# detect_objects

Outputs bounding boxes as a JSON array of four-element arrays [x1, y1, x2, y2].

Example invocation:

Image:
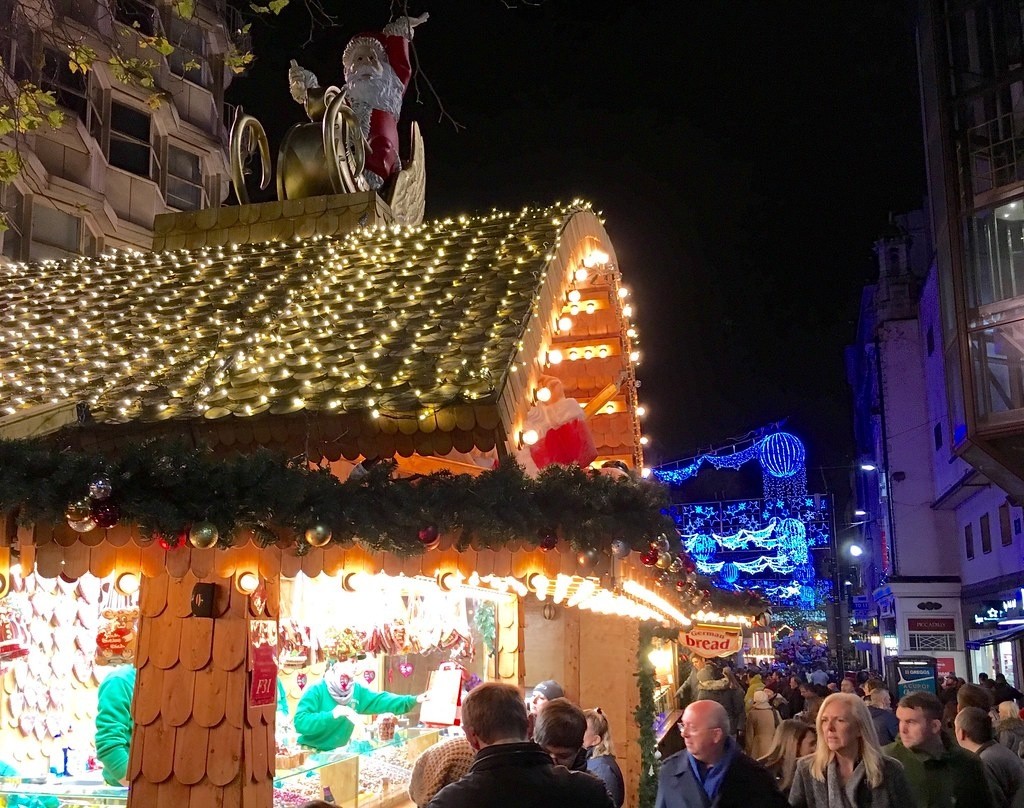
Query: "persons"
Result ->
[[405, 677, 625, 808], [651, 700, 794, 808], [292, 662, 432, 753], [672, 630, 1024, 755], [754, 718, 818, 798], [786, 693, 915, 808], [275, 674, 290, 754], [880, 690, 993, 808], [94, 666, 135, 788], [950, 707, 1024, 808]]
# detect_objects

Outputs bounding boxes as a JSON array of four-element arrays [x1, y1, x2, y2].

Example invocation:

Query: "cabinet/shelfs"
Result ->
[[0, 783, 129, 808], [273, 725, 467, 808], [652, 685, 676, 731]]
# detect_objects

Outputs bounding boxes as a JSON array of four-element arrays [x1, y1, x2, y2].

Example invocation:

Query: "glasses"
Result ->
[[677, 722, 723, 736]]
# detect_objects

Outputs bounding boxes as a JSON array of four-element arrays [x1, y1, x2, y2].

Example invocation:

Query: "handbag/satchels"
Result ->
[[419, 662, 464, 727]]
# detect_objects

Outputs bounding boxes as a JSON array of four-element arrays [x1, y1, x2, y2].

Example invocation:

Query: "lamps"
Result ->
[[529, 573, 549, 592], [442, 572, 460, 591], [345, 573, 363, 592], [517, 250, 653, 479], [119, 573, 138, 594], [240, 572, 259, 592]]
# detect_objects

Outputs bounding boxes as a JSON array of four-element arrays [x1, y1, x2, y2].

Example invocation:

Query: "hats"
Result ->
[[696, 665, 714, 683], [764, 681, 777, 695], [409, 737, 477, 808], [533, 680, 564, 702], [946, 675, 959, 682]]
